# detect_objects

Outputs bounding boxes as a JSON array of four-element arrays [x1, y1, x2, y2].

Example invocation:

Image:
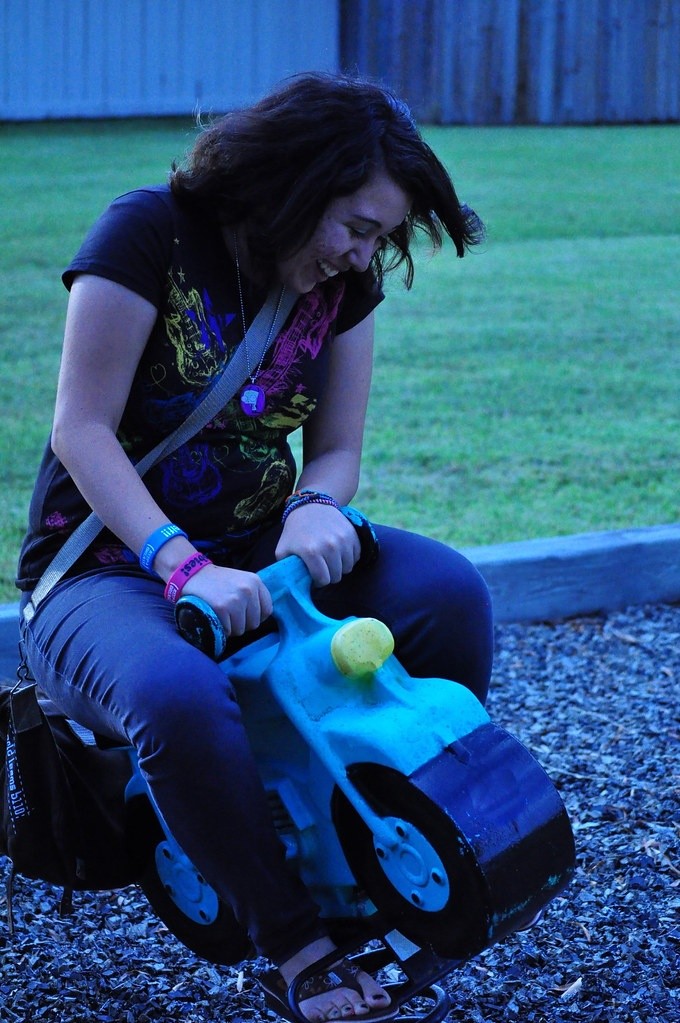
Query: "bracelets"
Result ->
[[281, 489, 339, 525], [139, 522, 188, 579], [164, 553, 213, 605]]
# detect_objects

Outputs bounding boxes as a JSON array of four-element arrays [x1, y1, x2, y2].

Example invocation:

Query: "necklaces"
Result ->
[[234, 235, 288, 418]]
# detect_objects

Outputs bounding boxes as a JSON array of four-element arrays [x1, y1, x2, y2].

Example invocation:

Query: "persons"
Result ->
[[16, 72, 497, 1023]]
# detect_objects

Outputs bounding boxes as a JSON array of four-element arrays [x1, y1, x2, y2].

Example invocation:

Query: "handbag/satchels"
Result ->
[[0, 670, 140, 890]]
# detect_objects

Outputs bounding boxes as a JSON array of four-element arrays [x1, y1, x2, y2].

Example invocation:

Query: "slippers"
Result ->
[[256, 956, 399, 1023]]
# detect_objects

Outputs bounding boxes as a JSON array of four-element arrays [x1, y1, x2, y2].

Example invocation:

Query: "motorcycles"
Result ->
[[0, 505, 577, 1018]]
